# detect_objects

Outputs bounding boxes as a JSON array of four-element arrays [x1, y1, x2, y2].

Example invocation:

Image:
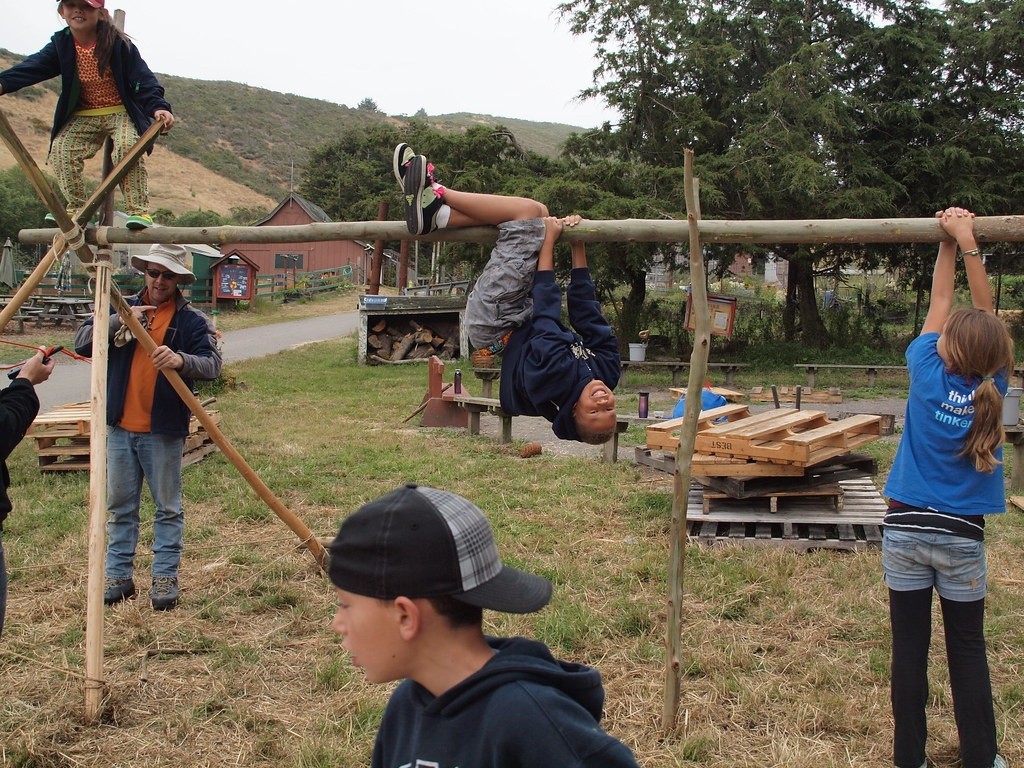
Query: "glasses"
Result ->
[[145, 268, 176, 280]]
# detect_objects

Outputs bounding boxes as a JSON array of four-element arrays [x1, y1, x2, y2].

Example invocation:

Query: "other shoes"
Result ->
[[895, 757, 927, 767], [961, 754, 1006, 768]]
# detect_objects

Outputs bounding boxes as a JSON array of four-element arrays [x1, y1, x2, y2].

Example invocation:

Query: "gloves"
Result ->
[[114, 321, 148, 346]]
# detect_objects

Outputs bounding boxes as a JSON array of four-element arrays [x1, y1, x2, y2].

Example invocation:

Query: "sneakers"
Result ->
[[152, 576, 178, 610], [392, 143, 415, 193], [45, 211, 76, 227], [405, 155, 446, 236], [104, 577, 135, 604], [126, 214, 154, 229]]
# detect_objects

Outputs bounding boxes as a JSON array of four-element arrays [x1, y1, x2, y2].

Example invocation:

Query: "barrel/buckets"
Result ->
[[1001, 388, 1022, 425], [629, 343, 646, 362]]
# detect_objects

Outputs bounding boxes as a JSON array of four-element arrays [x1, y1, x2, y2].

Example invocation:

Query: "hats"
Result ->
[[131, 244, 195, 285], [329, 483, 552, 614], [56, 0, 105, 8]]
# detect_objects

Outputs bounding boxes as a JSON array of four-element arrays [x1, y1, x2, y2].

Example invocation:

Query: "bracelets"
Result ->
[[962, 248, 980, 257]]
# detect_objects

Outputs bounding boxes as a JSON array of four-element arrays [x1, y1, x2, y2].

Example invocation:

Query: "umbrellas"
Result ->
[[0, 236, 17, 301], [55, 249, 72, 296]]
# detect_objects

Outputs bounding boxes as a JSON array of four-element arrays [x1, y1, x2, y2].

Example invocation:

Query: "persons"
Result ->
[[883, 206, 1015, 768], [2, 0, 174, 229], [1, 343, 63, 640], [393, 142, 621, 445], [328, 483, 639, 767], [75, 244, 223, 611]]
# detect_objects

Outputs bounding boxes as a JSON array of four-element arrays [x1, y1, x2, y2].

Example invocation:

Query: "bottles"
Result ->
[[638, 392, 650, 418]]
